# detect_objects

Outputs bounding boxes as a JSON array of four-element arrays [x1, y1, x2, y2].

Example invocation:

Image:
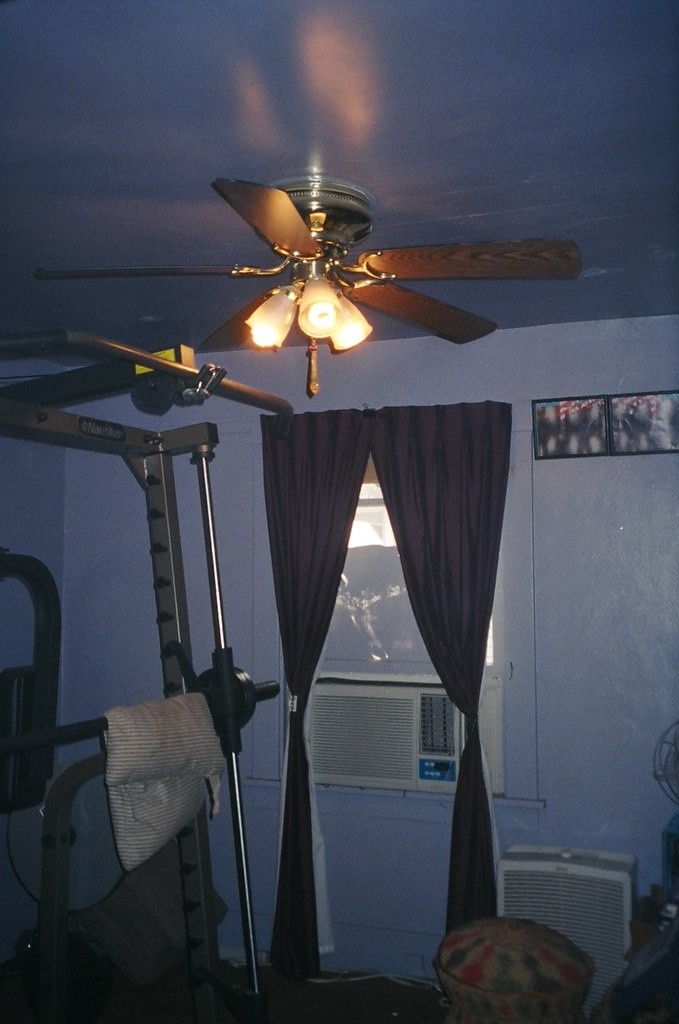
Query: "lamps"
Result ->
[[245, 277, 373, 352]]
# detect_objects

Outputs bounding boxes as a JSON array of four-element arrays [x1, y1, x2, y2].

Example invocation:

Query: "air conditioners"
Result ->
[[305, 679, 506, 797]]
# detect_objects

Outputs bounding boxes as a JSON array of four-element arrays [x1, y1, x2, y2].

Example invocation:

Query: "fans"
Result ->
[[654, 718, 679, 804], [32, 176, 582, 346]]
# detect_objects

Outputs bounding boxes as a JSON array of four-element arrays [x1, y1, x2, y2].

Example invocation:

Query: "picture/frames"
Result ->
[[608, 389, 679, 456], [532, 394, 610, 460]]
